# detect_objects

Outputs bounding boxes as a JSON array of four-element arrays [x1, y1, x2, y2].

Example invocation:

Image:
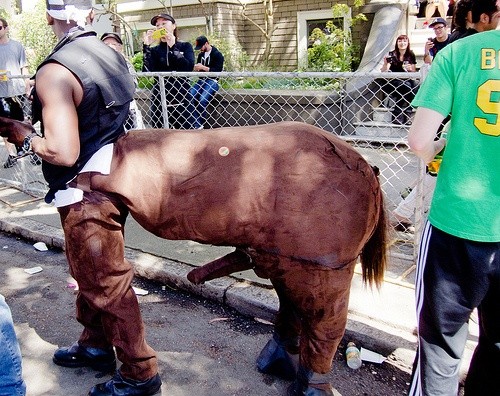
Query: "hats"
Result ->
[[150, 14, 175, 26], [46, 0, 93, 28], [100, 33, 123, 45], [194, 36, 207, 50], [428, 17, 447, 28]]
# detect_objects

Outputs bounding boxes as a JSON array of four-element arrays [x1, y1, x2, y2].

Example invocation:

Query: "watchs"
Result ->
[[23, 132, 38, 154]]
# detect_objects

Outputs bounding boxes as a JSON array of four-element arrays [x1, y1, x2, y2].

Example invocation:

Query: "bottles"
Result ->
[[346, 342, 362, 370]]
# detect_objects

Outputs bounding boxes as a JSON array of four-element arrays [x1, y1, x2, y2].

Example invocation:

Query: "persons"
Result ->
[[1, 19, 41, 168], [0, 295, 27, 396], [382, 0, 500, 396], [100, 14, 224, 130], [3, 0, 162, 396]]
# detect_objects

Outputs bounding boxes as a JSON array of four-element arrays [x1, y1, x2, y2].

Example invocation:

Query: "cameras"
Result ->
[[386, 54, 398, 64]]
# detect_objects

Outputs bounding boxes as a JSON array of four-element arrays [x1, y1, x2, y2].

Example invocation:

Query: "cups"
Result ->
[[403, 61, 409, 72]]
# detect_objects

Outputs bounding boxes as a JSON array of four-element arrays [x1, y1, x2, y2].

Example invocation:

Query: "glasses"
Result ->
[[433, 25, 445, 30], [0, 25, 7, 30]]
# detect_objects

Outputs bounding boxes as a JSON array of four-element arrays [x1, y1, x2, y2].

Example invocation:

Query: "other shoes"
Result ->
[[3, 155, 17, 168], [89, 369, 161, 396], [30, 154, 41, 166], [53, 340, 116, 374]]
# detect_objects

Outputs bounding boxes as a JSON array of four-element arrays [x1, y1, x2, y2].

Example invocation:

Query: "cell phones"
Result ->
[[151, 28, 166, 39]]
